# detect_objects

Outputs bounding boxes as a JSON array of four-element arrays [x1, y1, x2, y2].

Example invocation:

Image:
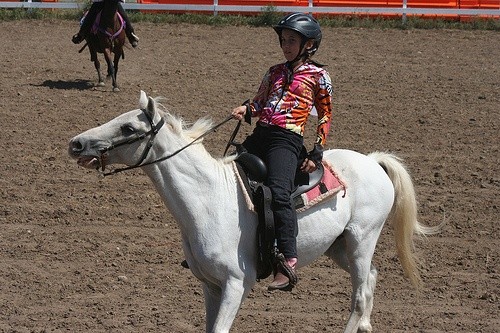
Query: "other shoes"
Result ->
[[128, 33, 139, 47], [268, 266, 296, 291], [72, 37, 80, 44]]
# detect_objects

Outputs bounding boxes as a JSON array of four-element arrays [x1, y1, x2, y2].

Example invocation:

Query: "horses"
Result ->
[[83, 0, 125, 93], [67, 91, 452, 333]]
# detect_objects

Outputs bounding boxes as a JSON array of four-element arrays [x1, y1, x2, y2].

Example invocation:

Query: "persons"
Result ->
[[180, 12, 333, 291], [72, 0, 139, 48]]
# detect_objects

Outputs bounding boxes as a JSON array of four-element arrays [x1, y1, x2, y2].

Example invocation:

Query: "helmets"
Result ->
[[272, 12, 323, 58]]
